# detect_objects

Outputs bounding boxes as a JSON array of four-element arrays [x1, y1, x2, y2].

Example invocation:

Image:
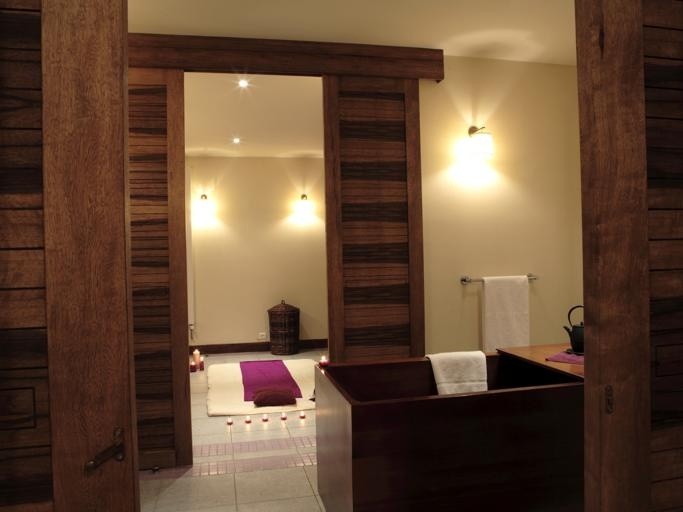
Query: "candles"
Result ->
[[190, 347, 204, 374], [318, 355, 328, 368], [226, 410, 306, 426]]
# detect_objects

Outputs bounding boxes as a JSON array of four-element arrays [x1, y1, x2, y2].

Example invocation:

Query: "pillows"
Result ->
[[251, 386, 296, 405]]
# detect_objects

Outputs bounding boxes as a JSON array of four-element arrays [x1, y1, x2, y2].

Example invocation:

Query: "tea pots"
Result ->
[[563, 305, 585, 353]]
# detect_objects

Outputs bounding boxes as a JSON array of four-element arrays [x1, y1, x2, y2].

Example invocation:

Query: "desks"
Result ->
[[496, 343, 584, 379]]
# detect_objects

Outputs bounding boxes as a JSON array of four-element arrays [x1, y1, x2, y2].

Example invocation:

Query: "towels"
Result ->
[[425, 351, 488, 396], [481, 275, 529, 355]]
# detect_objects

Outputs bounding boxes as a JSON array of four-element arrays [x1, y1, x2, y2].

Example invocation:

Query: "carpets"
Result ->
[[207, 359, 319, 416]]
